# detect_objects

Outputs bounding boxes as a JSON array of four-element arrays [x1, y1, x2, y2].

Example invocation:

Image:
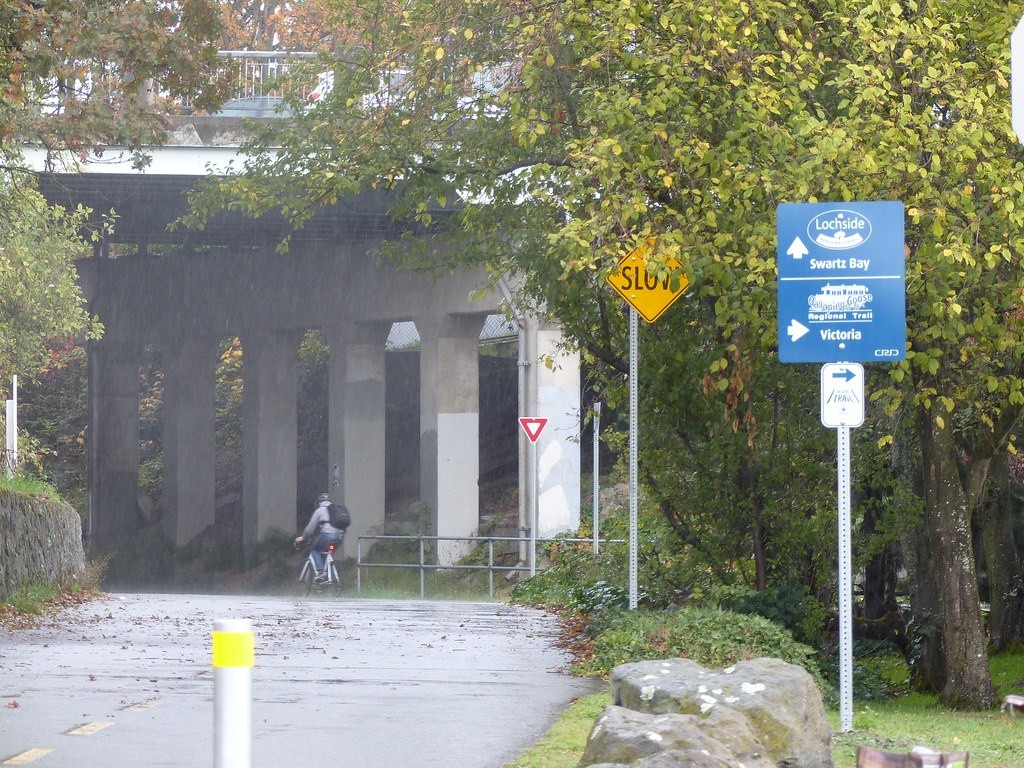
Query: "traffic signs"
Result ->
[[775, 200, 907, 363]]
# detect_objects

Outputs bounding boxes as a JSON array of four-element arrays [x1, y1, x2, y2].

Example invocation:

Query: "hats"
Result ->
[[317, 492, 331, 501]]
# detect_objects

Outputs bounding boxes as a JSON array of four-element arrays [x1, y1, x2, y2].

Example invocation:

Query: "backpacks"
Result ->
[[318, 503, 351, 529]]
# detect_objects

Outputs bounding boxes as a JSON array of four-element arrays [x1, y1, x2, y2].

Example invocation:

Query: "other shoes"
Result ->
[[314, 571, 327, 581]]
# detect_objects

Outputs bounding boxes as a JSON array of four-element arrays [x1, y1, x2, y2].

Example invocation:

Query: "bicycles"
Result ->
[[291, 538, 346, 597]]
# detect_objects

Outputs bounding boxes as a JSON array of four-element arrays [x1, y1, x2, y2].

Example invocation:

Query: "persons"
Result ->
[[295, 493, 347, 585]]
